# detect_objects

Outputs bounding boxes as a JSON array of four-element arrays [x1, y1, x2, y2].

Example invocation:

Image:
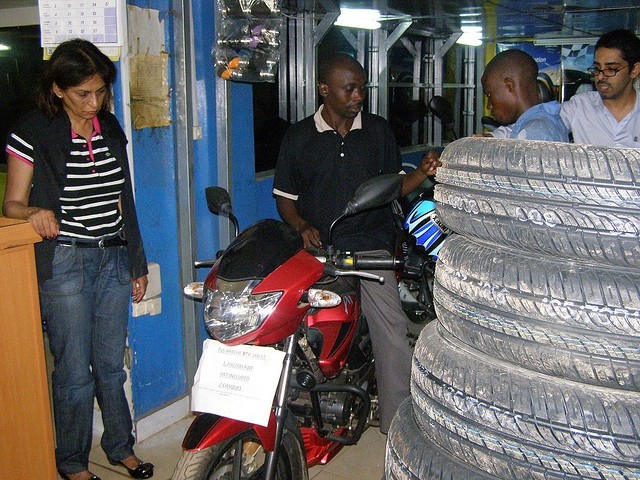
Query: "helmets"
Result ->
[[402, 197, 453, 265]]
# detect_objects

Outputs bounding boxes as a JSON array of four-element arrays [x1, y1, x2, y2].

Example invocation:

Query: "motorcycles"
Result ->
[[399, 186, 456, 325], [170, 176, 436, 480]]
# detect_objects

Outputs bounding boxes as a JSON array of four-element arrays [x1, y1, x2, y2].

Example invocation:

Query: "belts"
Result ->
[[47, 237, 128, 250]]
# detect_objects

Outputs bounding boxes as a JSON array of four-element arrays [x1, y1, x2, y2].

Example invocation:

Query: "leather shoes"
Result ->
[[110, 456, 154, 479]]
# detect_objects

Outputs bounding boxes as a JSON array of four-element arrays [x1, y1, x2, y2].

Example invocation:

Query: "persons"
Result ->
[[481, 48, 570, 146], [3, 38, 155, 480], [471, 28, 639, 147], [273, 52, 442, 436]]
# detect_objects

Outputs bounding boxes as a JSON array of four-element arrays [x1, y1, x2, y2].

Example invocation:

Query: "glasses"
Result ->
[[585, 65, 627, 77]]
